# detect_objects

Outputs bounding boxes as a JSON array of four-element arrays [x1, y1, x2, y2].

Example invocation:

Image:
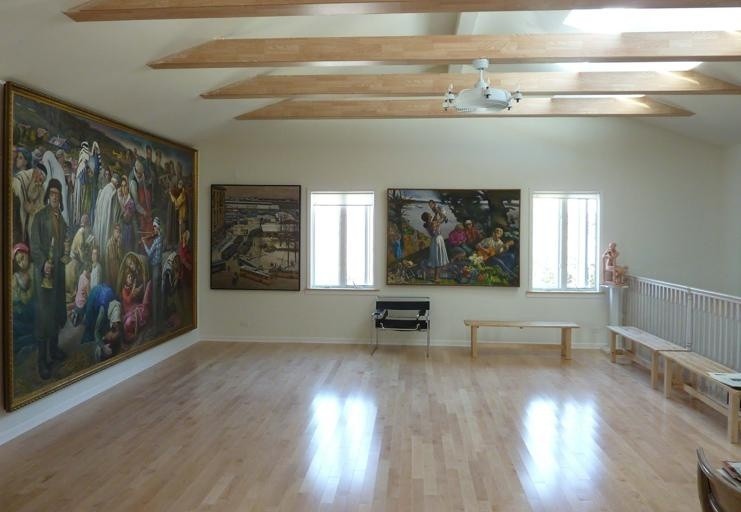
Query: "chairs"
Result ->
[[692, 448, 741, 512], [370, 298, 433, 358]]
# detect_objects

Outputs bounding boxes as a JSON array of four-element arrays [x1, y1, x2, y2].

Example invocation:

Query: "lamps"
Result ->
[[440, 57, 523, 116]]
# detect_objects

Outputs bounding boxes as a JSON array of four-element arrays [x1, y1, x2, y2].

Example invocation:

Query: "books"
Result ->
[[717, 459, 741, 488], [707, 370, 741, 388]]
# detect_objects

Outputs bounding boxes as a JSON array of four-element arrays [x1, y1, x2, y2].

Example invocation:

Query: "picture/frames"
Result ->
[[208, 181, 303, 295], [1, 77, 200, 415], [384, 185, 525, 288]]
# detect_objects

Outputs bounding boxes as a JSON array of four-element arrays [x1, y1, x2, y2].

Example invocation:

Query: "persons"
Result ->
[[390, 199, 514, 271]]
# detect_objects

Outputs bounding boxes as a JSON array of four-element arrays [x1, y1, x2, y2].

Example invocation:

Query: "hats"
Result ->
[[43, 178, 64, 212], [465, 220, 472, 224]]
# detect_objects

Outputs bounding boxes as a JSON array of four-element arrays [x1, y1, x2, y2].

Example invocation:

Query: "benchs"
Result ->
[[462, 319, 581, 358], [607, 323, 741, 443]]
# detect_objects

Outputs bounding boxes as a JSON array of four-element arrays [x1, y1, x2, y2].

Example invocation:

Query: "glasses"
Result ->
[[49, 191, 59, 197]]
